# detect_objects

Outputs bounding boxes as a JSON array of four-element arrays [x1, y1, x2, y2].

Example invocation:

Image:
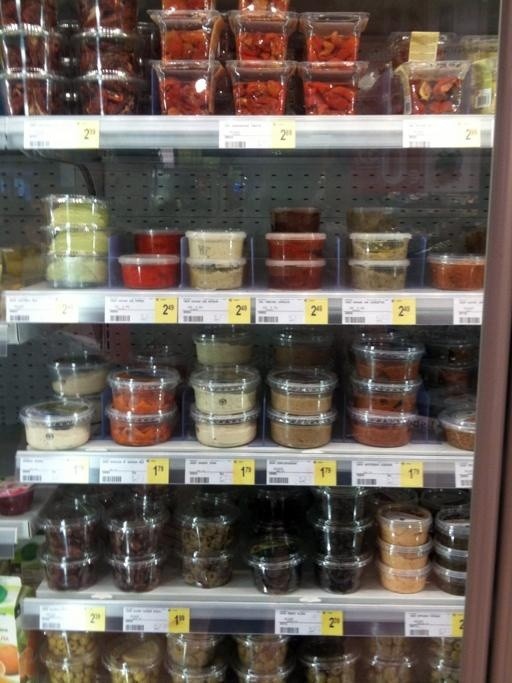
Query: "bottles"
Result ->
[[270, 206, 321, 233]]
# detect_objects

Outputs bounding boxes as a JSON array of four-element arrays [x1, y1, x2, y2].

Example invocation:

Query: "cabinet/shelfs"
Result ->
[[3, 0, 512, 683]]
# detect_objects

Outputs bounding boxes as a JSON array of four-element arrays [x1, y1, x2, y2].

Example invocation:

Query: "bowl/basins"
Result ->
[[33, 495, 106, 560], [310, 486, 377, 527], [69, 0, 150, 115], [264, 231, 328, 260], [1, 0, 72, 116], [43, 629, 96, 655], [105, 367, 183, 415], [375, 534, 433, 569], [250, 486, 304, 521], [180, 554, 234, 589], [0, 473, 36, 516], [346, 405, 418, 448], [187, 404, 262, 449], [102, 501, 171, 557], [101, 545, 169, 593], [374, 502, 433, 546], [183, 228, 248, 258], [297, 641, 363, 683], [164, 632, 227, 667], [37, 644, 103, 683], [439, 406, 477, 451], [271, 327, 336, 368], [366, 488, 419, 512], [175, 503, 240, 555], [422, 336, 476, 401], [305, 512, 376, 557], [243, 535, 311, 596], [40, 196, 111, 228], [228, 670, 297, 683], [130, 228, 185, 255], [162, 673, 229, 683], [102, 404, 178, 448], [128, 485, 177, 510], [423, 663, 461, 683], [99, 635, 165, 682], [310, 551, 375, 594], [421, 488, 469, 512], [353, 332, 427, 384], [191, 326, 256, 365], [231, 634, 292, 670], [266, 405, 338, 450], [426, 251, 486, 291], [2, 246, 44, 277], [435, 506, 470, 550], [36, 544, 100, 592], [190, 488, 244, 510], [431, 558, 467, 597], [44, 352, 109, 397], [49, 395, 102, 436], [66, 484, 116, 510], [17, 398, 96, 450], [249, 522, 299, 540], [266, 365, 339, 416], [185, 257, 249, 290], [374, 553, 433, 594], [44, 254, 107, 287], [117, 253, 180, 289], [364, 636, 419, 662], [348, 231, 412, 262], [264, 258, 327, 289], [346, 259, 410, 292], [427, 636, 465, 668], [433, 538, 469, 572], [360, 662, 418, 683], [46, 223, 109, 257], [188, 369, 261, 415], [350, 368, 423, 412]]
